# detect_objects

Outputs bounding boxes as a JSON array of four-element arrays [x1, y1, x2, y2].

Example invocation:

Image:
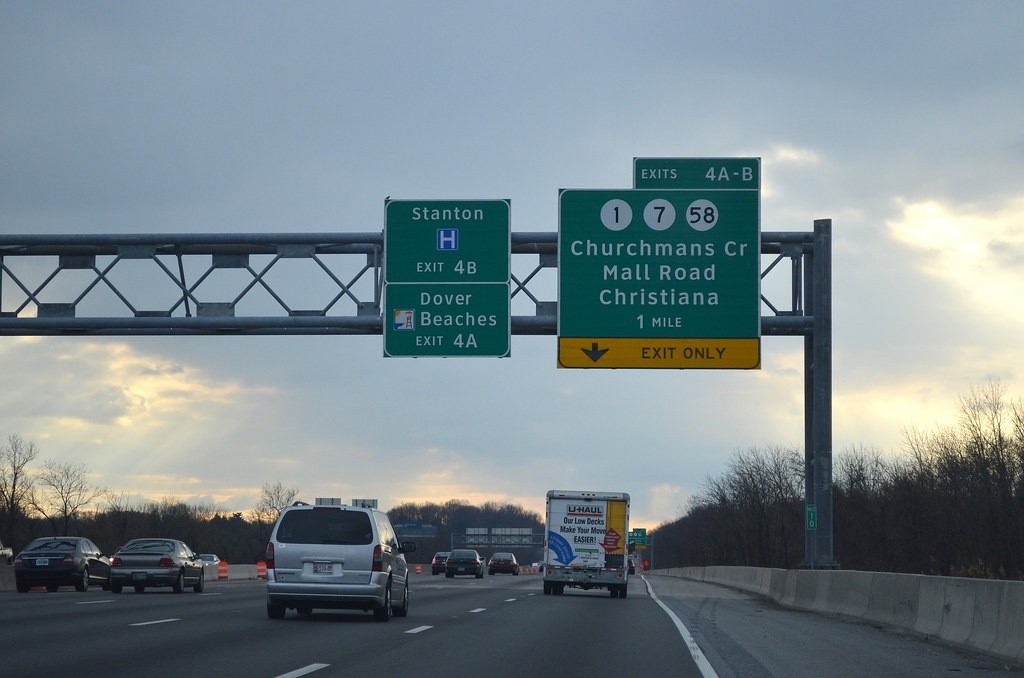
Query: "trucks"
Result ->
[[541, 489, 636, 599]]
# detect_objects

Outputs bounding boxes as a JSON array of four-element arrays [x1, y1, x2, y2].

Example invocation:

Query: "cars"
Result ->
[[489, 552, 520, 576], [14, 536, 112, 593], [531, 562, 542, 571], [628, 557, 636, 575], [432, 552, 451, 576], [443, 549, 487, 579], [109, 538, 206, 593], [199, 554, 220, 567], [0, 539, 14, 565]]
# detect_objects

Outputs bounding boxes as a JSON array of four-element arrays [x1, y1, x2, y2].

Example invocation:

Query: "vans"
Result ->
[[266, 501, 417, 622]]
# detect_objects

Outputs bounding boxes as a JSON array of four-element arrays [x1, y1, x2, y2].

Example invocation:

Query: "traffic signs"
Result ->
[[628, 532, 646, 549], [556, 189, 766, 372]]
